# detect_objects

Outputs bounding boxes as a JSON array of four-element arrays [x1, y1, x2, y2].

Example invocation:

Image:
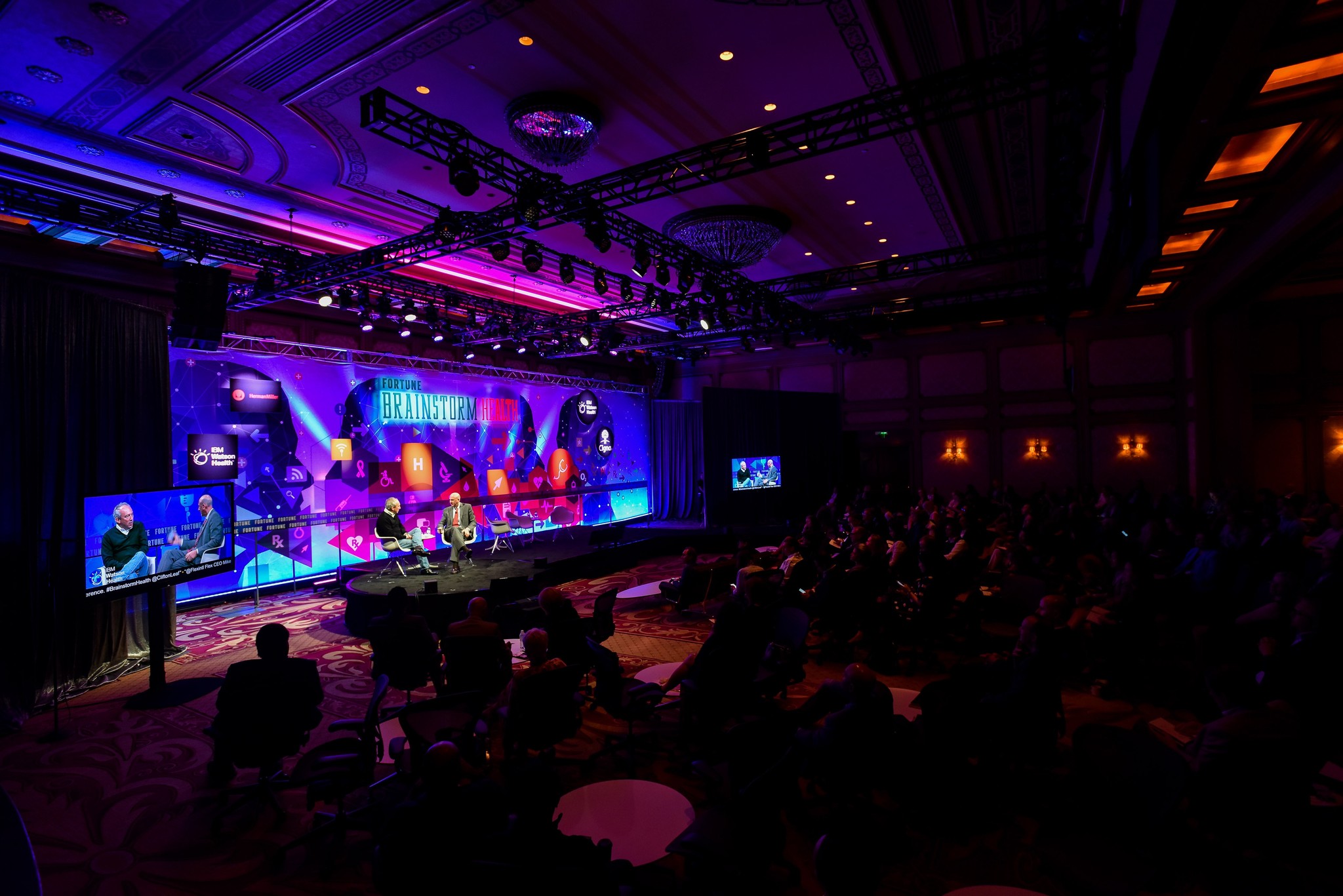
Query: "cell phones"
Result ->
[[896, 580, 904, 587]]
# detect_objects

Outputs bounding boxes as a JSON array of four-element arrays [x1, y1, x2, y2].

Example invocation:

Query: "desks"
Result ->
[[503, 638, 548, 664], [616, 575, 682, 599], [372, 717, 411, 766], [755, 547, 779, 553], [888, 687, 921, 720], [550, 779, 695, 868], [634, 662, 684, 696]]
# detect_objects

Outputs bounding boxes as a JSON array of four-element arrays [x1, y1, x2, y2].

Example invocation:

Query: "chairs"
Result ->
[[216, 489, 1343, 896]]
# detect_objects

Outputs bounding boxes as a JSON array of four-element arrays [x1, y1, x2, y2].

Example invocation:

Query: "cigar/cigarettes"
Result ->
[[509, 642, 515, 646]]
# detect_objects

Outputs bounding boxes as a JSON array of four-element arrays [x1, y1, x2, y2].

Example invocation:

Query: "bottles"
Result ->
[[527, 512, 530, 517], [494, 517, 498, 521], [534, 511, 538, 518], [519, 629, 526, 650], [427, 526, 430, 537]]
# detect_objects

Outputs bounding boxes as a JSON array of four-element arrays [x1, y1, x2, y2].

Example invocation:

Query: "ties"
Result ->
[[453, 508, 459, 526]]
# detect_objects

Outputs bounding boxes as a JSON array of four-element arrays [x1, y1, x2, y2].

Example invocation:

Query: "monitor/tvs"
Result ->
[[81, 482, 235, 607], [730, 455, 782, 492]]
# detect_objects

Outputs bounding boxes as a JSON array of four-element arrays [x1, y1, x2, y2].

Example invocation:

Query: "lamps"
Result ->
[[163, 86, 799, 396]]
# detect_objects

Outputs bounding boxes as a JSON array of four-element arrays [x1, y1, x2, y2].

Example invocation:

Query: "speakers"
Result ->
[[652, 358, 675, 398], [169, 261, 230, 351]]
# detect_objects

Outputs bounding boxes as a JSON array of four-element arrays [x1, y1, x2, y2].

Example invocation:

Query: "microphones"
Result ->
[[394, 518, 396, 520], [455, 511, 457, 514], [202, 524, 204, 527], [126, 534, 129, 537]]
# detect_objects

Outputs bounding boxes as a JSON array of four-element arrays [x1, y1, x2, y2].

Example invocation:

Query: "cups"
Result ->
[[424, 532, 427, 536], [530, 516, 534, 520], [422, 533, 423, 537]]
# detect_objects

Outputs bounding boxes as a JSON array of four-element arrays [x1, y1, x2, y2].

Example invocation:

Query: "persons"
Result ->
[[376, 496, 439, 575], [441, 597, 514, 699], [100, 500, 150, 582], [504, 626, 570, 760], [200, 622, 325, 789], [537, 586, 582, 654], [736, 460, 752, 487], [370, 587, 444, 693], [753, 459, 778, 486], [437, 492, 477, 575], [157, 494, 224, 573], [653, 476, 1342, 896]]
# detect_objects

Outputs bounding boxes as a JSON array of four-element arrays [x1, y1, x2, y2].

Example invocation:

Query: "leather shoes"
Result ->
[[421, 568, 438, 574], [465, 549, 473, 559], [412, 548, 431, 557], [451, 566, 460, 574]]
